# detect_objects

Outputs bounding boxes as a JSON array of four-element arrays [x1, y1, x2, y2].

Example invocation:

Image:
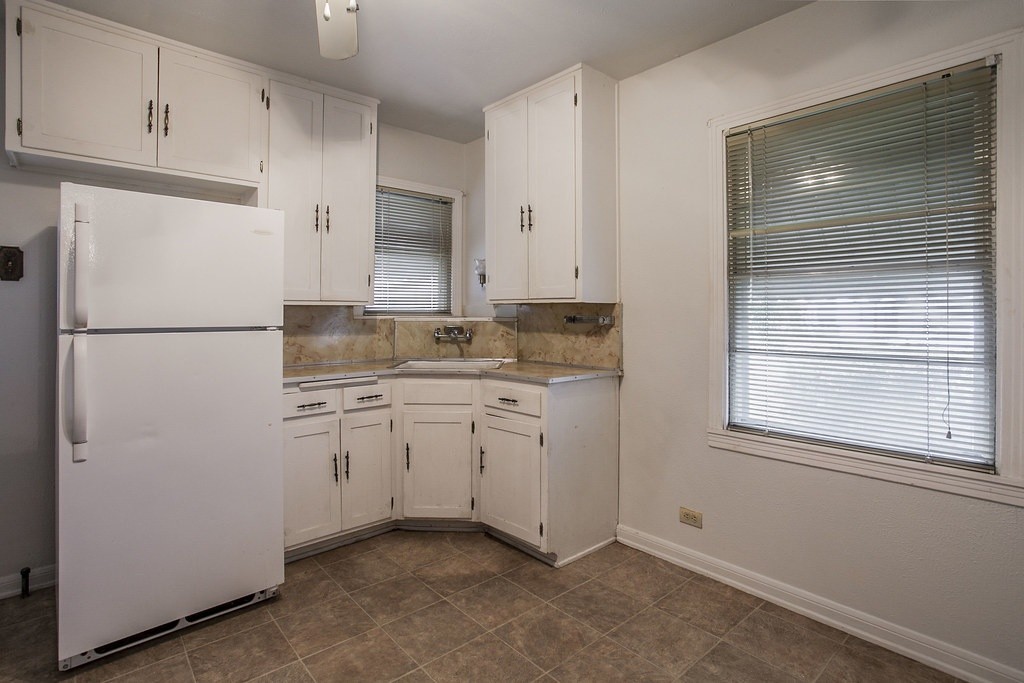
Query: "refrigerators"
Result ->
[[57, 180, 285, 673]]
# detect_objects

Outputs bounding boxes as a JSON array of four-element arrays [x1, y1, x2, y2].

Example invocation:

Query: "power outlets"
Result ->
[[680, 507, 702, 529]]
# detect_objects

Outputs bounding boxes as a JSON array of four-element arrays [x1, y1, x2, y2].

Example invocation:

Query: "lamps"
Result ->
[[473, 258, 486, 288]]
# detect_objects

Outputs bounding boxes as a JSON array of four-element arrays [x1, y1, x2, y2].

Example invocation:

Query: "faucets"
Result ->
[[450, 330, 458, 340], [465, 328, 472, 339]]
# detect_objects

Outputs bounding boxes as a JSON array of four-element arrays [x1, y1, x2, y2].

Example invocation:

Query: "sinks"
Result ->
[[395, 359, 505, 368]]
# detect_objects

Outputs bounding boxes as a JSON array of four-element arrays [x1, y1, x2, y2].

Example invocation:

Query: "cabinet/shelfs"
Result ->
[[241, 74, 381, 305], [5, 0, 266, 200], [283, 360, 619, 569], [483, 62, 620, 304]]
